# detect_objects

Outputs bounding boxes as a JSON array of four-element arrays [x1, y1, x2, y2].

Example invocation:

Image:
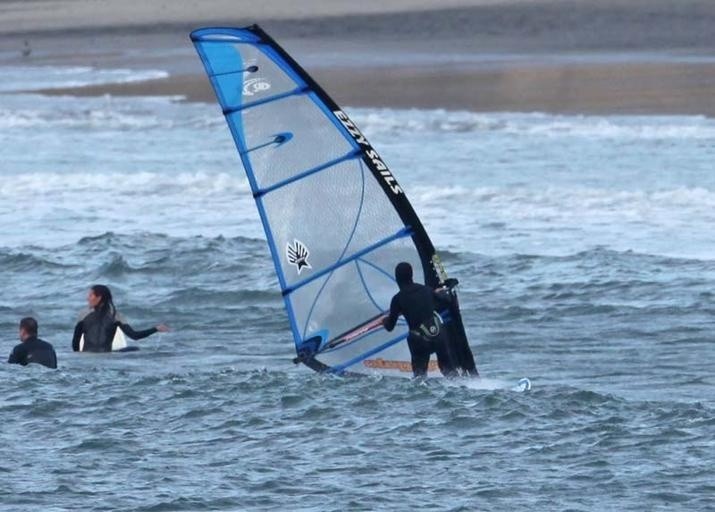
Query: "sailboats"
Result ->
[[187, 22, 532, 395]]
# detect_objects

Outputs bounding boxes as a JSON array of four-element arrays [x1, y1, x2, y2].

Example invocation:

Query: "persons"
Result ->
[[379, 261, 462, 380], [7, 317, 57, 369], [72, 285, 169, 353]]
